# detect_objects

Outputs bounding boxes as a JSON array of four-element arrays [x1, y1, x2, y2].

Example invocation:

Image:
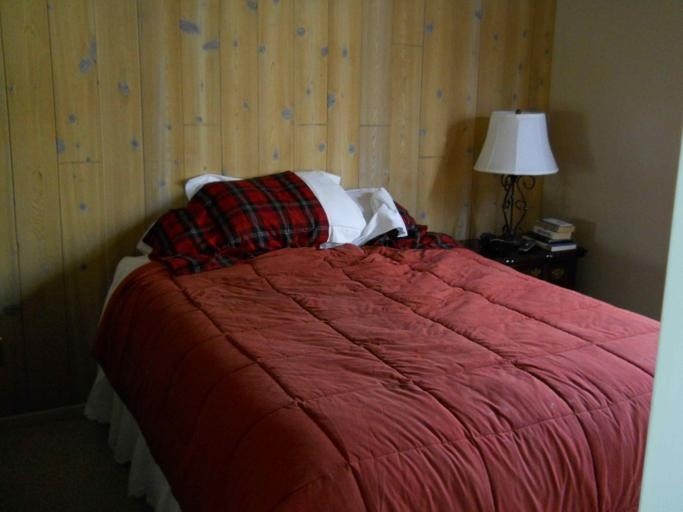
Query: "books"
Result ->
[[520, 218, 579, 252]]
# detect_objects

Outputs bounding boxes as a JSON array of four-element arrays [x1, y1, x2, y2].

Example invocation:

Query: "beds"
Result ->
[[81, 229, 661, 512]]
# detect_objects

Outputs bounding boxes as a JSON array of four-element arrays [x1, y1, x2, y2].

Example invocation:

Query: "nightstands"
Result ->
[[459, 237, 589, 292]]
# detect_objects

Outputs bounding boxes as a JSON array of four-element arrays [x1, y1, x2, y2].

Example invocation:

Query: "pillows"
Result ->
[[183, 169, 366, 245]]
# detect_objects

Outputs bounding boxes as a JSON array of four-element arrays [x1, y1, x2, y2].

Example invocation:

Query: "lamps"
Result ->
[[473, 109, 561, 252]]
